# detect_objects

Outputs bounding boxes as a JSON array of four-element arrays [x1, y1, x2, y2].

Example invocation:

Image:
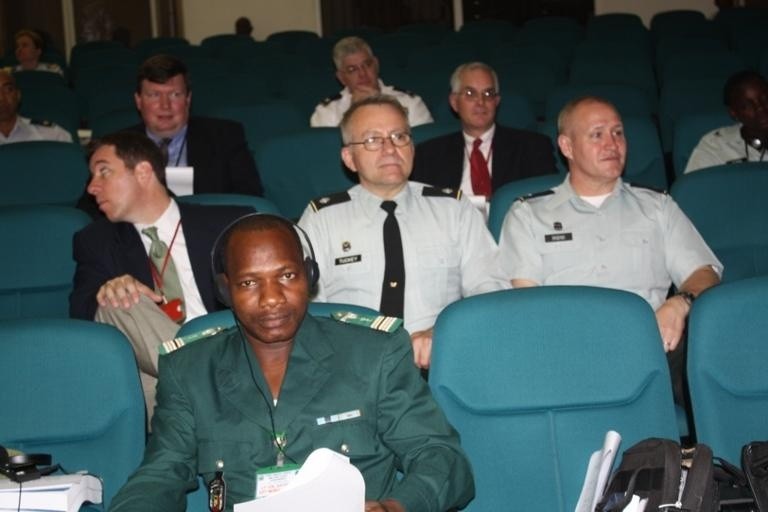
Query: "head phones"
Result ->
[[211, 211, 319, 308], [742, 125, 762, 150], [0, 445, 52, 474]]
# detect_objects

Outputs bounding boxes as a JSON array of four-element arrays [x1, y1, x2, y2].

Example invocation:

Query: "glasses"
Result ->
[[342, 129, 411, 152], [339, 56, 375, 72], [449, 87, 499, 100]]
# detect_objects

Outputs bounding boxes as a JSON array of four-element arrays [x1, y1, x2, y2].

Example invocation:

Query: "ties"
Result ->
[[142, 227, 189, 326], [379, 199, 406, 328], [468, 138, 495, 199], [154, 135, 173, 184]]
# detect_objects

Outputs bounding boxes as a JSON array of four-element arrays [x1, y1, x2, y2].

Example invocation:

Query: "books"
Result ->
[[0, 472, 102, 512]]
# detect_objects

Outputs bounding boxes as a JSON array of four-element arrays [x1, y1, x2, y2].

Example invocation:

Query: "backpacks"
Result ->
[[592, 438, 767, 511]]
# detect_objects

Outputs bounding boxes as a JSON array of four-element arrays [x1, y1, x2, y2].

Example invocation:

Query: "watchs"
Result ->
[[669, 288, 696, 306]]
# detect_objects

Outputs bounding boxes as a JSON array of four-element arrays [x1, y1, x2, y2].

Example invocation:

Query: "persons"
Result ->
[[2, 31, 62, 75], [306, 35, 437, 129], [66, 134, 244, 427], [78, 56, 263, 211], [107, 216, 473, 512], [233, 15, 255, 36], [0, 68, 75, 146], [496, 97, 731, 358], [684, 66, 768, 173], [410, 63, 558, 201], [293, 96, 497, 376]]
